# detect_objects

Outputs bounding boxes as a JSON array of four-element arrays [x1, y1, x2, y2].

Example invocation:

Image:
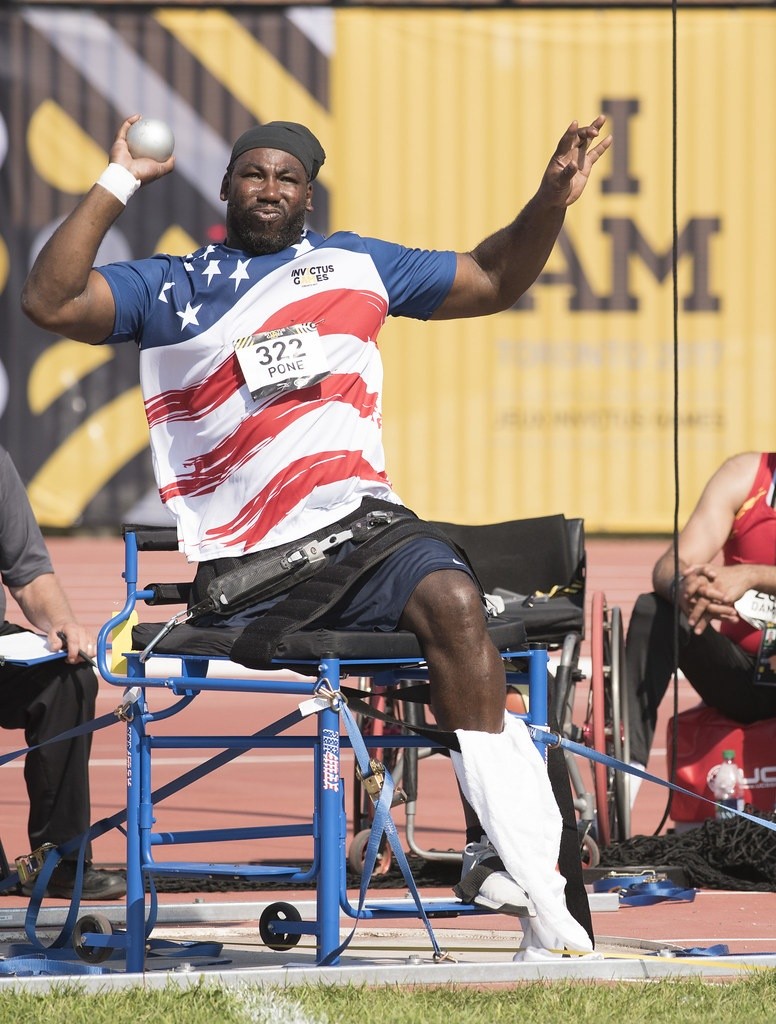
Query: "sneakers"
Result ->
[[454, 835, 534, 917]]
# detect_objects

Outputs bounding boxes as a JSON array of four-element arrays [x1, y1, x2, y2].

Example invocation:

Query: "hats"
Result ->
[[227, 121, 327, 181]]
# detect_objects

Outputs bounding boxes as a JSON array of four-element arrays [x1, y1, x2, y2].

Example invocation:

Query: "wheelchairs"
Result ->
[[350, 512, 634, 847]]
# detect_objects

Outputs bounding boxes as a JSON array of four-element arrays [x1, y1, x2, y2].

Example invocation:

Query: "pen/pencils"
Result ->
[[56, 632, 97, 668]]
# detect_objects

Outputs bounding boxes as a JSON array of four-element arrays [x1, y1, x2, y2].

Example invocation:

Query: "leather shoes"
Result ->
[[22, 859, 127, 900]]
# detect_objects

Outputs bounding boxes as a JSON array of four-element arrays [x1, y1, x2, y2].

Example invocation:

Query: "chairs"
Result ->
[[94, 514, 584, 972]]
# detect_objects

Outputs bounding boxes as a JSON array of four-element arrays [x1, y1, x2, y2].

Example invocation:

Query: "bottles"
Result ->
[[714, 751, 743, 821]]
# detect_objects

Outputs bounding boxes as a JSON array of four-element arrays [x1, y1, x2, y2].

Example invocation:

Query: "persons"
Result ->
[[0, 234, 126, 903], [620, 449, 774, 825], [18, 112, 612, 976]]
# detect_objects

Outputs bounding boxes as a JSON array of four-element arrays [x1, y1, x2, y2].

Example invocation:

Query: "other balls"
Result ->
[[126, 118, 173, 163]]
